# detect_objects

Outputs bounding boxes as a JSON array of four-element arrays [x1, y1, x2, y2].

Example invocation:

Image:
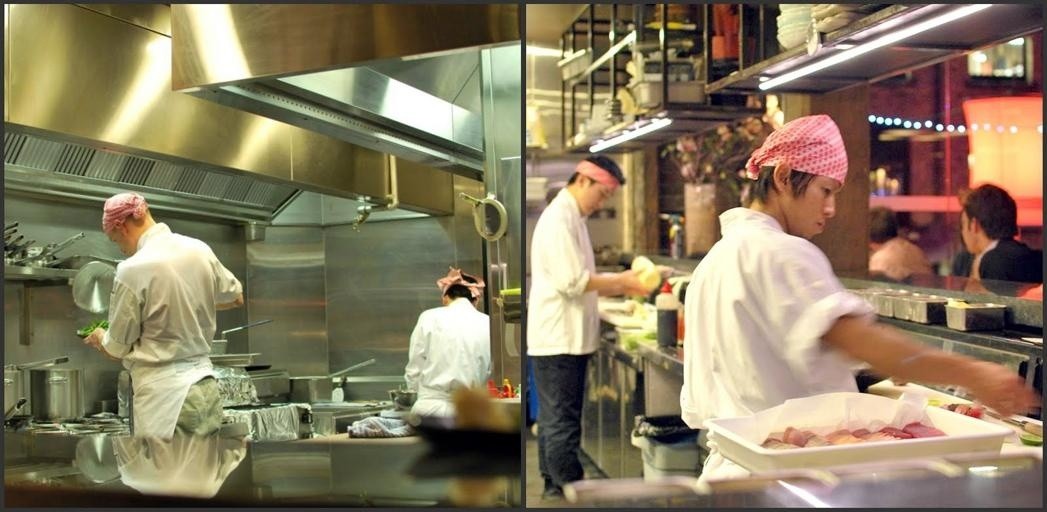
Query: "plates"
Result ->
[[246, 364, 271, 372], [774, 4, 814, 48]]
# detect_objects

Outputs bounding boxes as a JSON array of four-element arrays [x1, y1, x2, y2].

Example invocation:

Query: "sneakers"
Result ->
[[540, 487, 560, 501]]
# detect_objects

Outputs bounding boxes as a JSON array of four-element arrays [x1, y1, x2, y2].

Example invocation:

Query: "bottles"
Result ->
[[654, 280, 678, 350], [331, 376, 344, 402]]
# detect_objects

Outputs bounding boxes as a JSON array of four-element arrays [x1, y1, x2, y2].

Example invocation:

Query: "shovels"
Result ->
[[331, 376, 346, 403]]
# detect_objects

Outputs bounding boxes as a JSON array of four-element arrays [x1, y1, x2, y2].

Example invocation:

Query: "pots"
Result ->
[[3, 366, 85, 424], [210, 318, 272, 350], [289, 358, 376, 401], [73, 259, 119, 313], [3, 221, 85, 268]]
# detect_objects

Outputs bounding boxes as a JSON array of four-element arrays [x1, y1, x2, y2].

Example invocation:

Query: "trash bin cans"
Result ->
[[631, 414, 698, 481]]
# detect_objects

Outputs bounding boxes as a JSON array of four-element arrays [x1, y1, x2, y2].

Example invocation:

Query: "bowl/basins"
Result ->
[[851, 286, 1009, 332], [388, 388, 416, 410], [208, 353, 264, 367]]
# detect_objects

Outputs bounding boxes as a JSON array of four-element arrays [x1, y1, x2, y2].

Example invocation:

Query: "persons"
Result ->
[[526, 153, 653, 499], [857, 205, 937, 284], [79, 191, 258, 432], [404, 266, 496, 438], [959, 180, 1040, 290], [108, 431, 252, 497], [678, 113, 1041, 501]]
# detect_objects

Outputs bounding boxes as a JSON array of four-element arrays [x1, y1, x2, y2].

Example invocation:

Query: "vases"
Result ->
[[682, 180, 717, 256]]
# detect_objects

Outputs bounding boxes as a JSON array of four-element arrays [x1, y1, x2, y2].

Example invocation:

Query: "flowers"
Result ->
[[662, 114, 768, 198]]
